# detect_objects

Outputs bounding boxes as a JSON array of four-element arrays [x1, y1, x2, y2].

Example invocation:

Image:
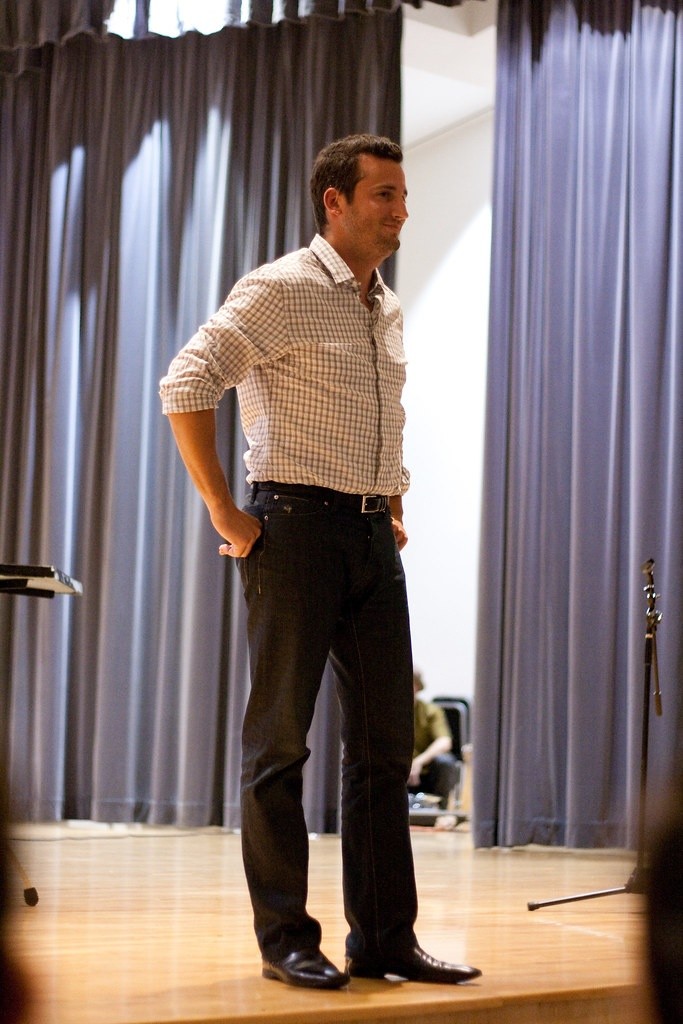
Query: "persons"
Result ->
[[157, 134, 480, 987], [405, 671, 458, 810]]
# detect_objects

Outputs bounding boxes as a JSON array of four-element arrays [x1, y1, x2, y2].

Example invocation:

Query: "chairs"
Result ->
[[408, 696, 470, 810]]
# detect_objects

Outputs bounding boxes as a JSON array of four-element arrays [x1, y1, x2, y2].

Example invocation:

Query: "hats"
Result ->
[[412, 670, 424, 691]]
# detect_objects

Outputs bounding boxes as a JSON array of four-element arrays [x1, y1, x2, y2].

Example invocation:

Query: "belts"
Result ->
[[249, 479, 388, 516]]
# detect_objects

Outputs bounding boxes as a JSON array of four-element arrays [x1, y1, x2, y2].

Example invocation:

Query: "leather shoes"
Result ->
[[261, 946, 349, 990], [344, 938, 482, 983]]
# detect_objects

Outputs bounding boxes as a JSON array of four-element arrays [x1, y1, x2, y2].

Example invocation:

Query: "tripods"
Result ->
[[525, 562, 664, 911]]
